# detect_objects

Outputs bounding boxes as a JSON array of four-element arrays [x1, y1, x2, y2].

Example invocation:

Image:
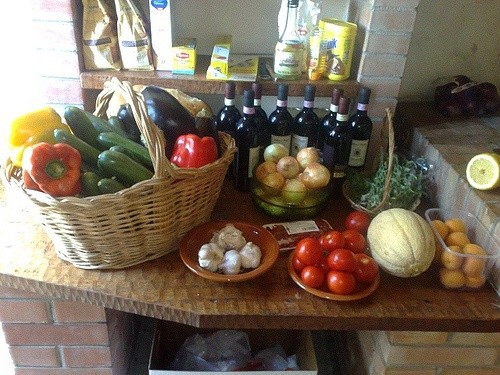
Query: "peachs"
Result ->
[[430, 218, 489, 288]]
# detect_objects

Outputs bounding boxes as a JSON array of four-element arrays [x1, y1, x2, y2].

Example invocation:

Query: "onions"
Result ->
[[253, 143, 331, 215]]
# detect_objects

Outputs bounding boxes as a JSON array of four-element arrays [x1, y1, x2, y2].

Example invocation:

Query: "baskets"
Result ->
[[5, 81, 237, 271], [343, 109, 420, 219]]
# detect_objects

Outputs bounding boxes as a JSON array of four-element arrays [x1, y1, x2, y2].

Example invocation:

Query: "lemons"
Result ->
[[465, 153, 500, 191]]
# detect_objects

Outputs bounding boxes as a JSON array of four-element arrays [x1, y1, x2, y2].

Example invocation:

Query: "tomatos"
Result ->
[[292, 228, 377, 295], [345, 210, 371, 234]]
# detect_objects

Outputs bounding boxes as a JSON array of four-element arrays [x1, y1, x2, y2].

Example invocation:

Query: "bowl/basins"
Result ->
[[252, 166, 331, 223]]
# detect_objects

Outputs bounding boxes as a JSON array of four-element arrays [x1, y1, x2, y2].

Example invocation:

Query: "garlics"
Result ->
[[198, 225, 262, 275]]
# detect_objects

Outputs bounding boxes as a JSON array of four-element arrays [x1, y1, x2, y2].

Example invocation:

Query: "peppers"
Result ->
[[10, 107, 83, 198], [171, 133, 218, 169]]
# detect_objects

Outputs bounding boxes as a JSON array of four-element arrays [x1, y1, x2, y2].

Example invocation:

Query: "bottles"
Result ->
[[348, 86, 374, 176], [234, 87, 260, 191], [322, 88, 352, 188], [216, 81, 243, 178], [269, 83, 291, 173], [242, 82, 268, 175], [272, 0, 304, 82], [291, 84, 320, 174]]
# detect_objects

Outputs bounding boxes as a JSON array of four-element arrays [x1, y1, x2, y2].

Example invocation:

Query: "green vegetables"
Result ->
[[343, 148, 423, 213]]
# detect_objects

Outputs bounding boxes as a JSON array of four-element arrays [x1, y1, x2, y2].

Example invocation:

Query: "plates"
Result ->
[[287, 243, 381, 301], [179, 218, 280, 283]]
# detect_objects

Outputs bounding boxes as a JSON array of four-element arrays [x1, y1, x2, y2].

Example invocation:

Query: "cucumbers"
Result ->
[[52, 105, 156, 196]]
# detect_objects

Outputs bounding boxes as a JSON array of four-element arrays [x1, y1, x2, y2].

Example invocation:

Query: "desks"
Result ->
[[0, 180, 500, 375]]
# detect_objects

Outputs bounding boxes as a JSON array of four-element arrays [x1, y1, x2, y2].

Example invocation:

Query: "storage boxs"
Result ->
[[146, 319, 320, 375], [425, 208, 500, 291], [149, 0, 233, 80]]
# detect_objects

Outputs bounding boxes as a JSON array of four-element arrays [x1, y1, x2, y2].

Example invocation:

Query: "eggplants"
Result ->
[[118, 86, 223, 157]]
